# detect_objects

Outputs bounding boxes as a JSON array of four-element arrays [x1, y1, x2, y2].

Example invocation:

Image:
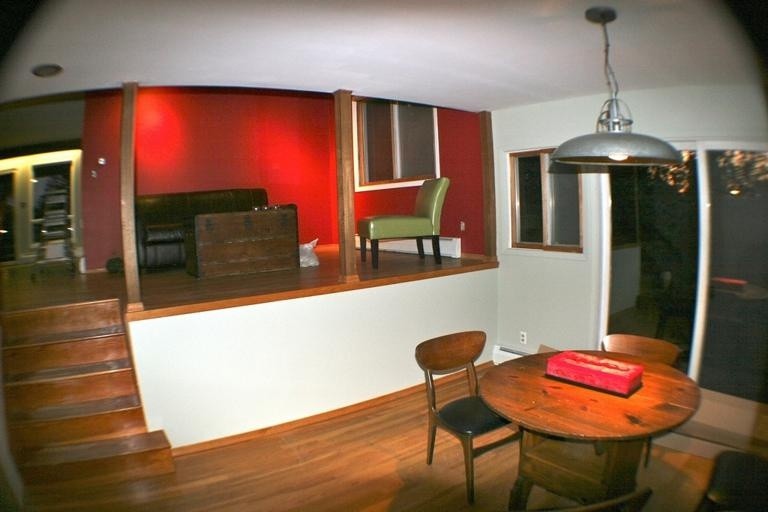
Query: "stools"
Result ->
[[697, 449, 768, 510]]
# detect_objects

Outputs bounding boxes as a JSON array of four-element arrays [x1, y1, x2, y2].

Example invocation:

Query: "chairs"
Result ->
[[547, 487, 653, 511], [654, 286, 690, 339], [600, 333, 683, 468], [414, 330, 524, 507]]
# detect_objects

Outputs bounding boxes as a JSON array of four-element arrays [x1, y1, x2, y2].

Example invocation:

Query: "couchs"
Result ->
[[355, 176, 451, 269], [134, 187, 267, 275]]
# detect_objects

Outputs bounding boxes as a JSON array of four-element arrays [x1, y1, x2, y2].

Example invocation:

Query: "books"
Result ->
[[545, 350, 646, 396], [34, 187, 73, 265]]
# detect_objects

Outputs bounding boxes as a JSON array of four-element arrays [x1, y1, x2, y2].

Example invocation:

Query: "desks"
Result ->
[[477, 350, 702, 512], [682, 281, 767, 374], [182, 203, 300, 279]]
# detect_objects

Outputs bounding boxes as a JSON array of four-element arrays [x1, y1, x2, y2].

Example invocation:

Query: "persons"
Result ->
[[569, 355, 631, 372]]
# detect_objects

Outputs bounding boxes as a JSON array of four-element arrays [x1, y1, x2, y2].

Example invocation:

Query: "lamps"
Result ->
[[548, 5, 683, 166]]
